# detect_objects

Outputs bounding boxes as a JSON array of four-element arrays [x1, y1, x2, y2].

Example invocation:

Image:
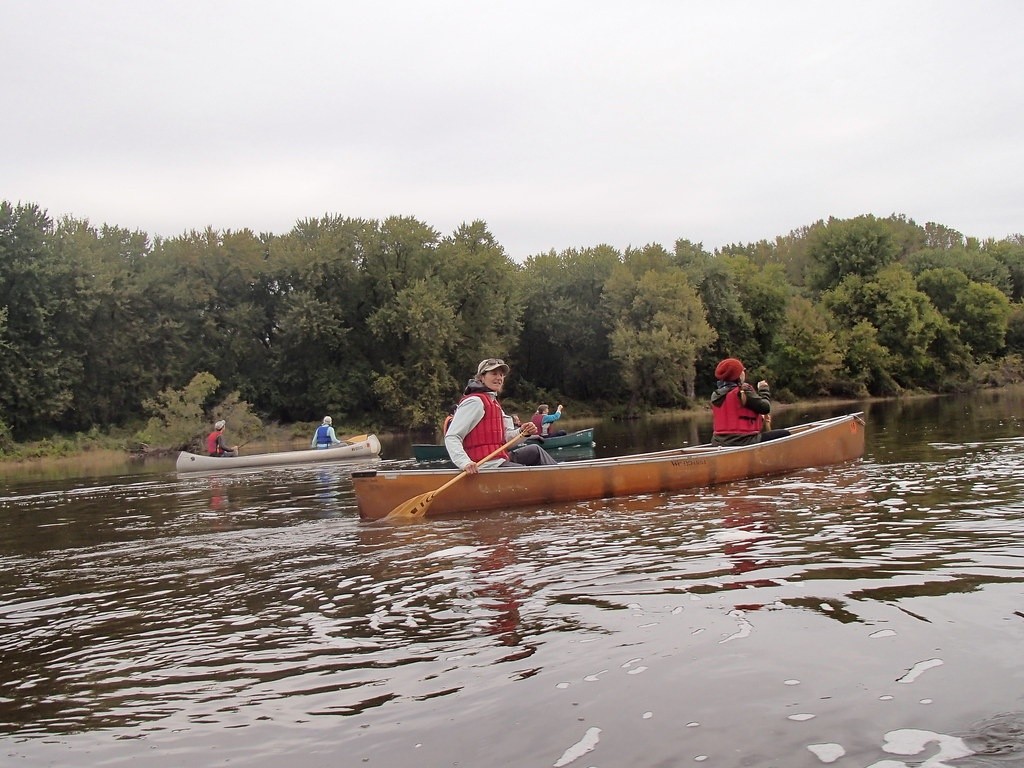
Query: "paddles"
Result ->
[[384, 429, 531, 524], [340, 435, 368, 443], [764, 414, 771, 432], [237, 435, 261, 449]]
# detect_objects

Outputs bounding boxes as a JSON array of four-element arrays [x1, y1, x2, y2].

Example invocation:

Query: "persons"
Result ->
[[443, 404, 458, 436], [531, 404, 567, 438], [711, 359, 791, 446], [208, 420, 238, 457], [445, 358, 558, 477], [312, 416, 348, 449], [510, 414, 544, 445]]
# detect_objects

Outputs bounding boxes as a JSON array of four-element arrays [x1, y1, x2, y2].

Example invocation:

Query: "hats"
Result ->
[[215, 420, 226, 430], [323, 416, 332, 424], [715, 359, 746, 382], [477, 358, 510, 374]]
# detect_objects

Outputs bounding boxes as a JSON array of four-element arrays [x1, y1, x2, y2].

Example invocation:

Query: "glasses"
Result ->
[[479, 359, 505, 373]]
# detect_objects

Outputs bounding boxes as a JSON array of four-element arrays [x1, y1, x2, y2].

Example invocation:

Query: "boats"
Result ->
[[350, 410, 867, 521], [175, 432, 381, 473], [410, 428, 594, 462]]
